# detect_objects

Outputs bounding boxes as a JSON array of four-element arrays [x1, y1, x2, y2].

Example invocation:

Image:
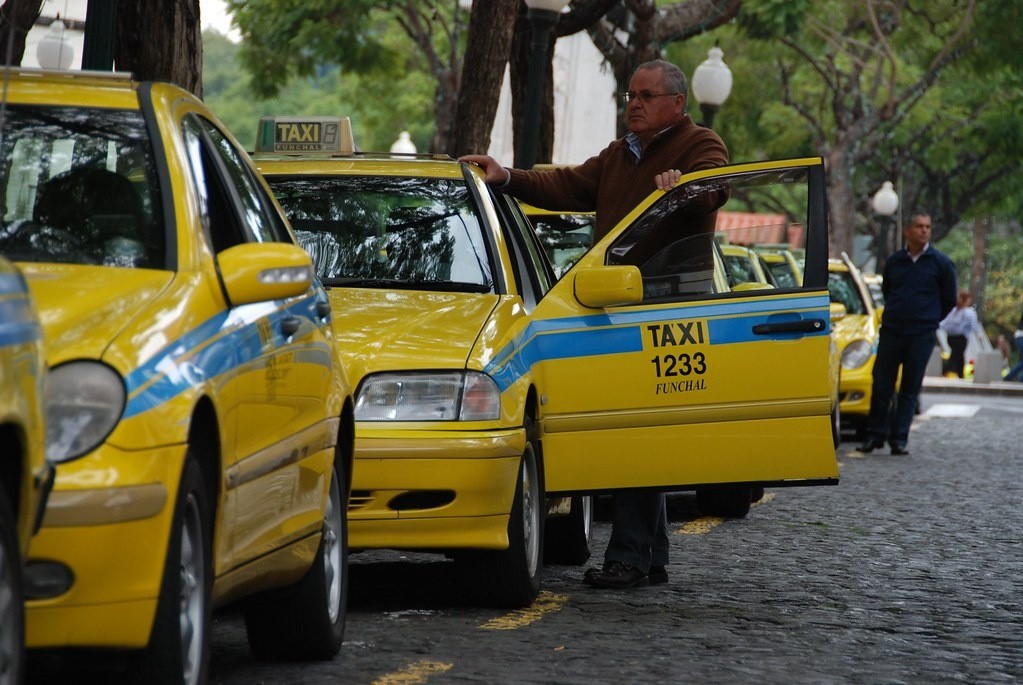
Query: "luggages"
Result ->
[[977, 338, 1004, 380]]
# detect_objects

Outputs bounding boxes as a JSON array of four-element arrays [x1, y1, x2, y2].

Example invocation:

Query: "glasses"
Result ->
[[618, 90, 679, 104]]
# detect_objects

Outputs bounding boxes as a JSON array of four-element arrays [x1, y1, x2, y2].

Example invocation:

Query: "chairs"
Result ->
[[386, 207, 449, 263], [33, 167, 142, 237]]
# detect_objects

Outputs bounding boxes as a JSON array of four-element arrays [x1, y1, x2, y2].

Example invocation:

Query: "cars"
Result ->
[[355, 163, 921, 444], [0, 33, 354, 685], [103, 115, 843, 612]]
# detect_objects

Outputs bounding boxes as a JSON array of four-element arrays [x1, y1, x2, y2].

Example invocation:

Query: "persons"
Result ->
[[853, 209, 1023, 456], [456, 60, 731, 589]]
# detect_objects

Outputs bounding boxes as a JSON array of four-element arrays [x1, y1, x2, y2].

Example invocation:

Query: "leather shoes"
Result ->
[[890, 443, 909, 455], [588, 562, 650, 590], [856, 435, 884, 453], [584, 561, 668, 586]]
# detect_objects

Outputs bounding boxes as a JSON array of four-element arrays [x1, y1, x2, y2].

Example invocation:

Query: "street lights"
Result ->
[[691, 46, 734, 132], [870, 181, 899, 279]]
